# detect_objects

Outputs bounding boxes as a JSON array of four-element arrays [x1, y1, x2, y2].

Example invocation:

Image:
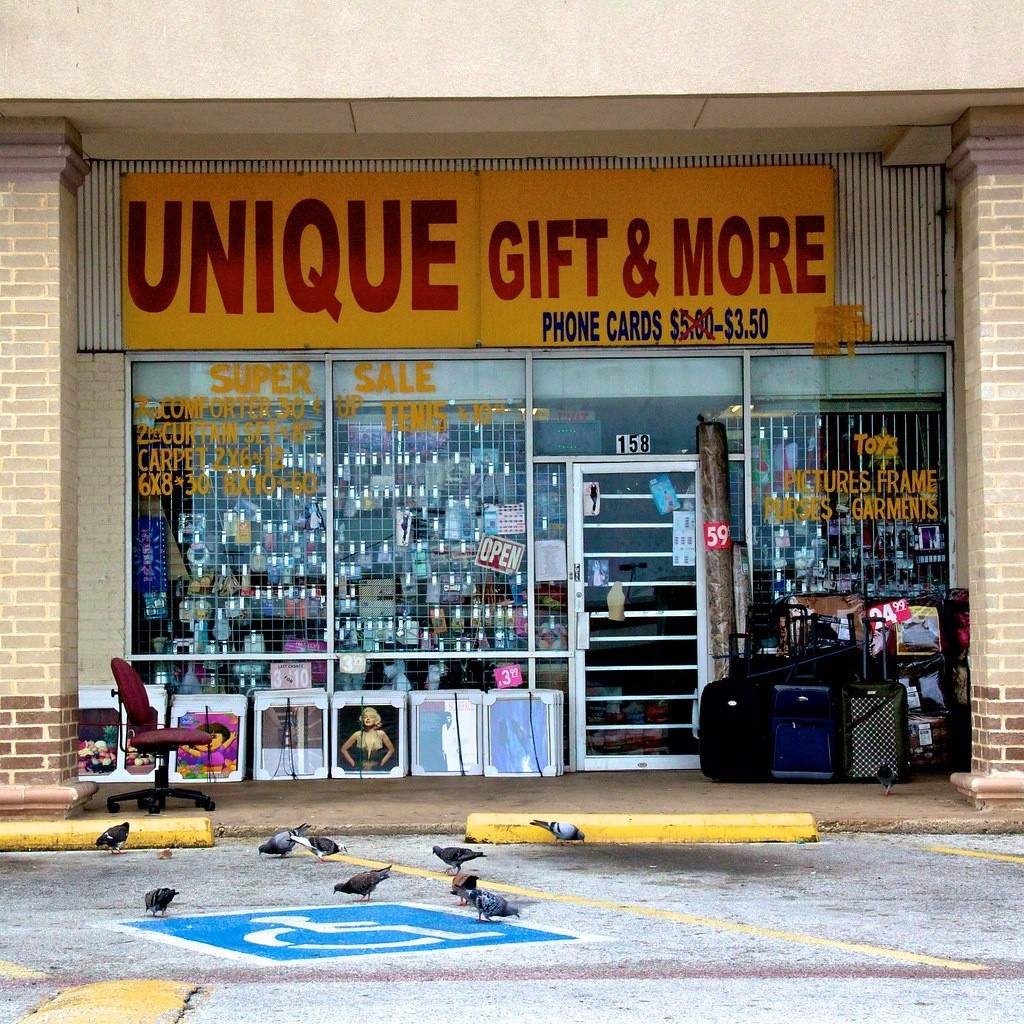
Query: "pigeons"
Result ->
[[451, 874, 519, 921], [433, 846, 487, 876], [334, 865, 392, 901], [877, 762, 898, 796], [257, 823, 347, 864], [530, 819, 585, 845], [145, 888, 180, 916], [95, 822, 131, 853]]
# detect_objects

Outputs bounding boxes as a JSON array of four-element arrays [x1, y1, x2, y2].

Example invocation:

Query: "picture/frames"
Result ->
[[409, 689, 486, 776], [253, 688, 329, 780], [484, 689, 564, 777], [168, 694, 248, 782], [77, 684, 167, 783], [331, 690, 407, 779]]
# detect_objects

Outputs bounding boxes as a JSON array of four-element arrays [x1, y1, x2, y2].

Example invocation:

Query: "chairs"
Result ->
[[107, 658, 215, 813]]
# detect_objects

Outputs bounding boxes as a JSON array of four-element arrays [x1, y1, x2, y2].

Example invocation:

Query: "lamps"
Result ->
[[719, 401, 756, 417], [518, 404, 538, 415]]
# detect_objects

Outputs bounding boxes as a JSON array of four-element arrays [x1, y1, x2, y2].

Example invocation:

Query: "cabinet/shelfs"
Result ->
[[722, 404, 944, 648], [132, 408, 578, 693]]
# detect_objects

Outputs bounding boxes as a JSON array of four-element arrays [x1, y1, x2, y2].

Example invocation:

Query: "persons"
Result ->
[[591, 561, 608, 587], [586, 482, 599, 515], [398, 513, 410, 546], [341, 707, 395, 770], [441, 712, 463, 772]]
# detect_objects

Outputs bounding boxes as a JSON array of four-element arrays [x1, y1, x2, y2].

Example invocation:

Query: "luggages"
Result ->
[[699, 589, 971, 785]]
